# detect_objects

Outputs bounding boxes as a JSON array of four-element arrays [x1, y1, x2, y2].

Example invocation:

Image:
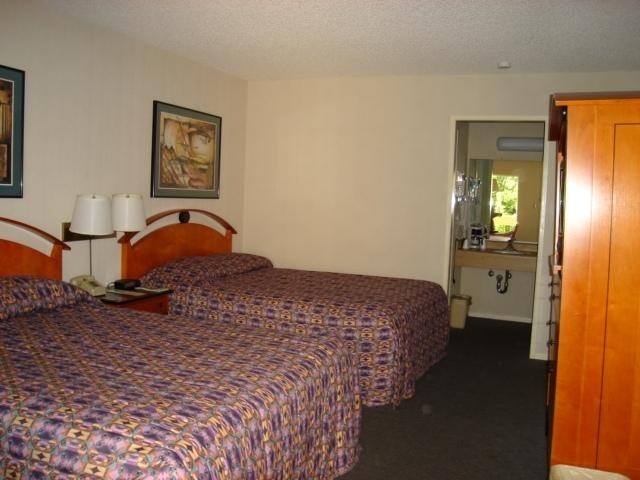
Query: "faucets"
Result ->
[[507, 238, 517, 251]]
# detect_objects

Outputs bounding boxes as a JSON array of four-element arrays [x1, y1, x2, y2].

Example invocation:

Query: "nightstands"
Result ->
[[102, 275, 170, 313]]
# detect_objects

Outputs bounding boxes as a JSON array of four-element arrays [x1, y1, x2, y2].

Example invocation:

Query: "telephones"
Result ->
[[70, 274, 106, 297]]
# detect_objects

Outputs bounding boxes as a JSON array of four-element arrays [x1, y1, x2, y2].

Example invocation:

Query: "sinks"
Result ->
[[495, 250, 528, 255]]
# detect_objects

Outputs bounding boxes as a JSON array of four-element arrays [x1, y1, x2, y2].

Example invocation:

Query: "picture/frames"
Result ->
[[0, 63, 30, 199], [149, 99, 223, 199]]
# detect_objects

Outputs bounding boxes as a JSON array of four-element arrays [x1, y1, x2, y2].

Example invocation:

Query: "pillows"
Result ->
[[0, 272, 105, 320], [148, 251, 273, 287]]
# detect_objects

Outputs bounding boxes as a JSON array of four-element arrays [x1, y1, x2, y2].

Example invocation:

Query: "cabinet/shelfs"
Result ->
[[547, 91, 640, 479]]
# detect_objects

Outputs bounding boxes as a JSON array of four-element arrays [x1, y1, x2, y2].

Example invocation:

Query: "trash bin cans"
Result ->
[[449, 294, 472, 329]]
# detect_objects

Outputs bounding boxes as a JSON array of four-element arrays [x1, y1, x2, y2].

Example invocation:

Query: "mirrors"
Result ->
[[467, 158, 542, 245]]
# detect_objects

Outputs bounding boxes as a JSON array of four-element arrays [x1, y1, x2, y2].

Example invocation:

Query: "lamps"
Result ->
[[61, 190, 147, 248], [496, 136, 545, 153]]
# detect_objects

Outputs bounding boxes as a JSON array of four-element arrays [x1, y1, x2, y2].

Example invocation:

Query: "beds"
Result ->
[[0, 217, 359, 478], [119, 209, 449, 406]]
